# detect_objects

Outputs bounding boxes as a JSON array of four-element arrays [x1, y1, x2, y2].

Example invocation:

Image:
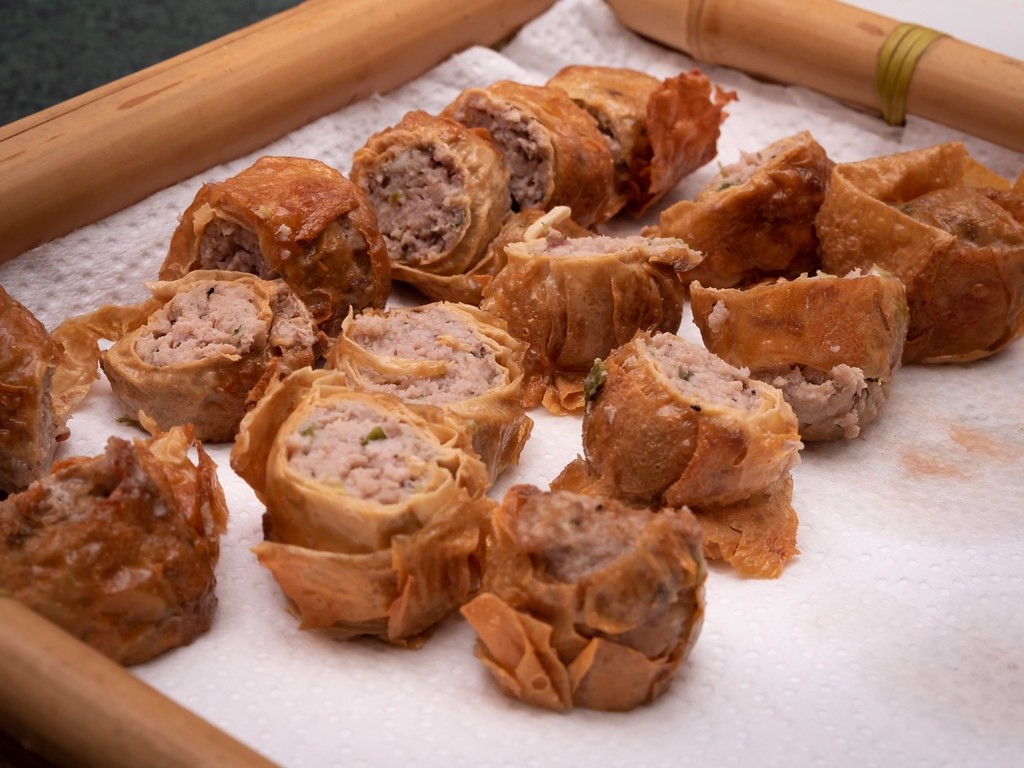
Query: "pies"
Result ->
[[0, 62, 1024, 715]]
